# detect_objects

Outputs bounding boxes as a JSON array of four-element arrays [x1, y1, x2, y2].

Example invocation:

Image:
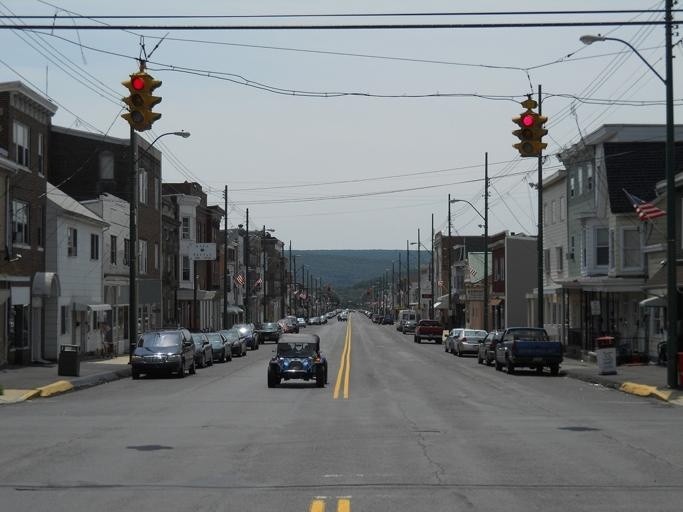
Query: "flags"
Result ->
[[626, 192, 667, 222], [233, 271, 245, 287]]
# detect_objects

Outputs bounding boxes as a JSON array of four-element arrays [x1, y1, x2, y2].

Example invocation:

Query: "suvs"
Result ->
[[267, 332, 327, 388], [130, 327, 195, 380], [257, 322, 283, 343], [231, 323, 259, 350]]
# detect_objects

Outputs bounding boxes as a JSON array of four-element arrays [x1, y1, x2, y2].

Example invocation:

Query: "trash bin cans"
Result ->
[[595, 336, 617, 376]]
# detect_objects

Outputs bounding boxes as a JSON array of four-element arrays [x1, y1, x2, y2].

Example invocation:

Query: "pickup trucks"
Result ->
[[413, 319, 444, 344], [491, 327, 563, 377]]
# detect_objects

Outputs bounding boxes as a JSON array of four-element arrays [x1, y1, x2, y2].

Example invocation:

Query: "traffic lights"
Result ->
[[519, 113, 538, 157], [120, 77, 134, 129], [129, 131, 190, 364], [127, 75, 148, 129], [510, 114, 524, 157], [534, 115, 548, 156], [146, 79, 162, 130]]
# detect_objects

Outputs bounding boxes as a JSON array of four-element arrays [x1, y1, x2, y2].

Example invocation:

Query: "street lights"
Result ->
[[449, 198, 490, 333], [359, 258, 409, 309], [409, 242, 434, 321], [280, 254, 345, 318], [245, 229, 275, 324], [578, 35, 679, 389]]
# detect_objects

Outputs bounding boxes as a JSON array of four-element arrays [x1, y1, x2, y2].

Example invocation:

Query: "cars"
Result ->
[[276, 308, 419, 334], [214, 329, 247, 357], [444, 328, 464, 353], [477, 329, 505, 366], [204, 333, 232, 363], [189, 333, 213, 369], [451, 329, 487, 357]]
